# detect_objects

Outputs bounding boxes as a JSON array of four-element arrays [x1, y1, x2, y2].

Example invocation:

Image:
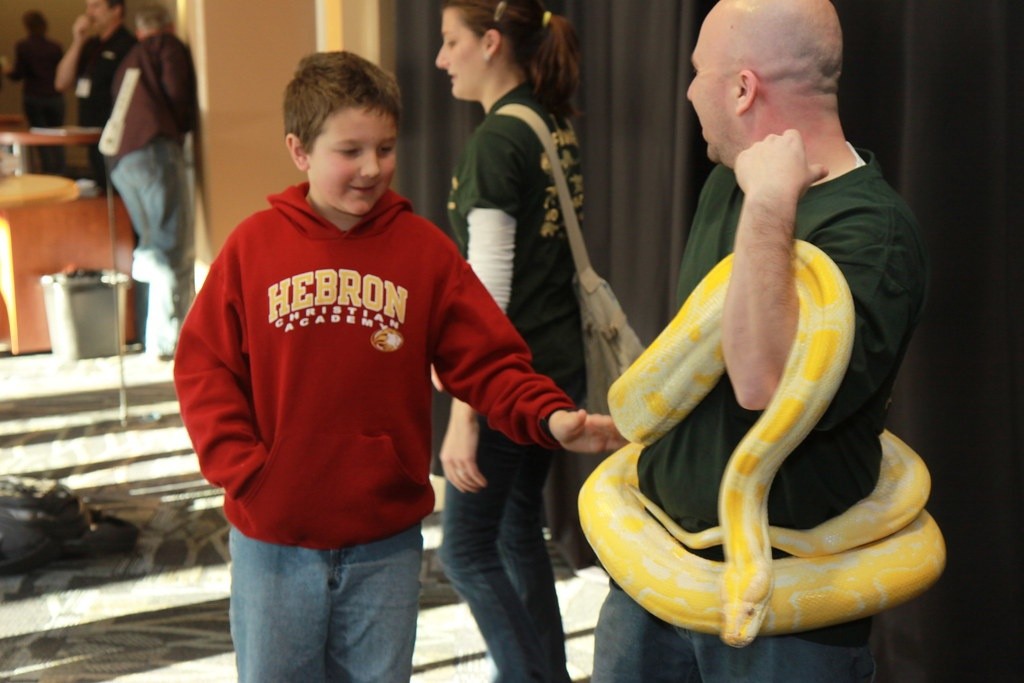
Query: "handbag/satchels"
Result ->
[[544, 265, 662, 575]]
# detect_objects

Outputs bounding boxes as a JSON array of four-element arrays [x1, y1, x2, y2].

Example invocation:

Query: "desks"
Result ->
[[1, 127, 147, 357]]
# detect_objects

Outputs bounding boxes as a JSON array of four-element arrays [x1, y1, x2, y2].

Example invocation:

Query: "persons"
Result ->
[[592, 0, 929, 683], [100, 5, 200, 360], [176, 51, 631, 683], [438, 0, 587, 683], [55, 0, 139, 198], [0, 10, 67, 177]]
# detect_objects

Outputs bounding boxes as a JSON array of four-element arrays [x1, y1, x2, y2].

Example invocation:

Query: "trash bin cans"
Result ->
[[41, 269, 129, 360]]
